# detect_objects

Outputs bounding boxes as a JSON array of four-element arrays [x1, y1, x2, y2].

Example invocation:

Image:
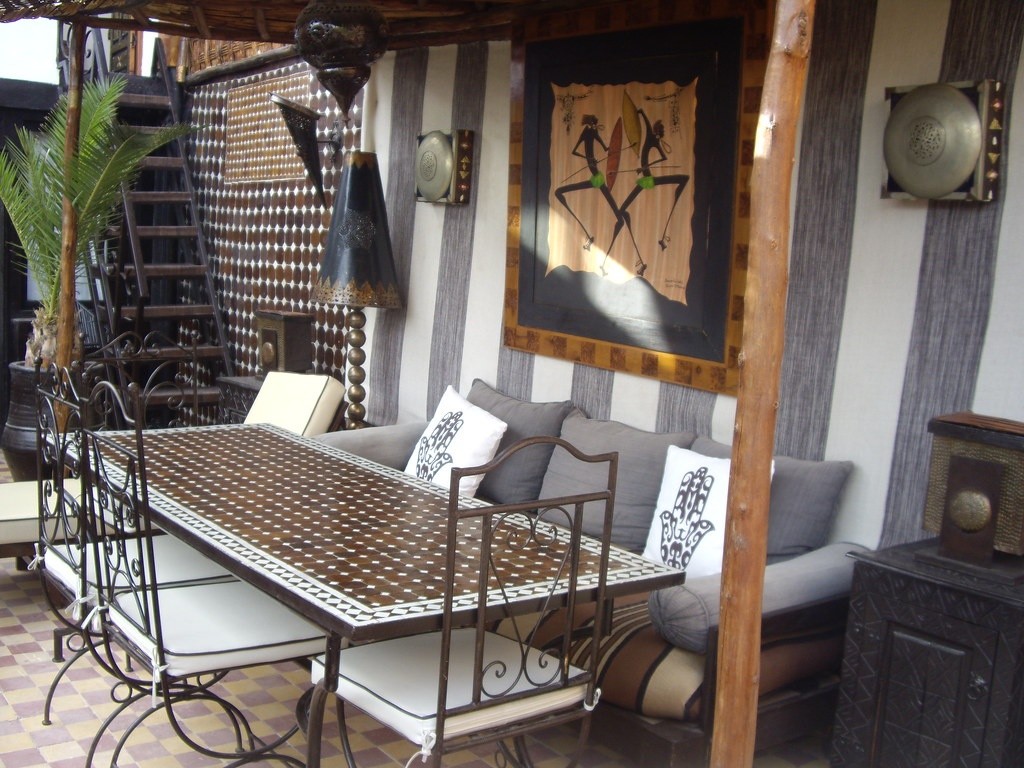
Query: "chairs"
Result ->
[[33, 354, 348, 767], [0, 370, 347, 563], [311, 434, 619, 768]]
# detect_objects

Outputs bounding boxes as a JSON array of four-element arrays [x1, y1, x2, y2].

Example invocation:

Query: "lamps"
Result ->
[[309, 150, 402, 432]]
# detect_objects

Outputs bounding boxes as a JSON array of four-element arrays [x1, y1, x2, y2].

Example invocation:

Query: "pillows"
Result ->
[[535, 407, 697, 554], [688, 434, 854, 567], [642, 444, 776, 579], [404, 385, 508, 499], [466, 378, 574, 514]]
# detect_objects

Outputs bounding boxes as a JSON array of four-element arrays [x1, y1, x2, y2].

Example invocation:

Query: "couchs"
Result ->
[[313, 423, 879, 768]]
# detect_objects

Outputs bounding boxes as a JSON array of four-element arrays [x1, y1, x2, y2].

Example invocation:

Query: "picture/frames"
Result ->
[[504, 0, 777, 400]]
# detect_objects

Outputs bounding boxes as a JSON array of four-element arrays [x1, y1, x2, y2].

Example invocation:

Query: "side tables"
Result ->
[[824, 539, 1024, 768], [215, 376, 264, 425]]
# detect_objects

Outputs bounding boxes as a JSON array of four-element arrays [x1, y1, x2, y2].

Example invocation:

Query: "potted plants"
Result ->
[[0, 72, 207, 483]]
